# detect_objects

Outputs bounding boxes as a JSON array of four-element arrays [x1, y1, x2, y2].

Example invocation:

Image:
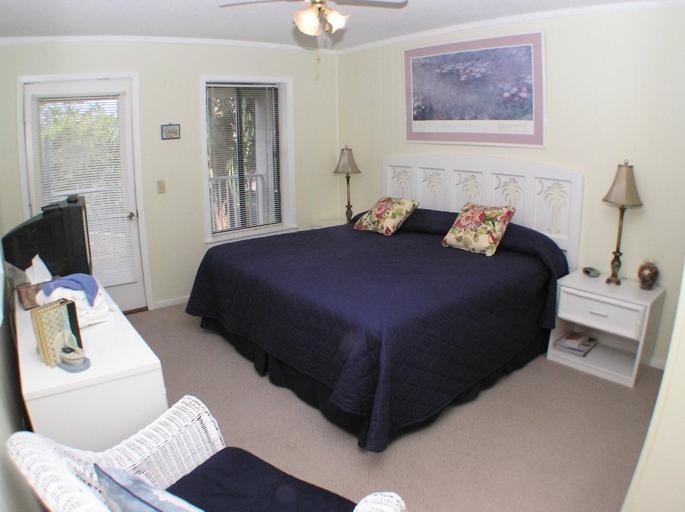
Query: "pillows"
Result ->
[[90, 461, 202, 512], [443, 200, 516, 255], [353, 193, 418, 235]]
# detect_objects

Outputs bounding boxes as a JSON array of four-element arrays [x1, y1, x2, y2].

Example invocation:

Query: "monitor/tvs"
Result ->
[[1, 196, 93, 277]]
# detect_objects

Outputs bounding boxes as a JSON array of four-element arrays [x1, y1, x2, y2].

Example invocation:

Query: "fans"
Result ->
[[216, 0, 408, 12]]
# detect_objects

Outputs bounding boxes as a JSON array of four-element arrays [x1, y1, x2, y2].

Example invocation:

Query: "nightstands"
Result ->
[[310, 217, 347, 231], [547, 267, 666, 387]]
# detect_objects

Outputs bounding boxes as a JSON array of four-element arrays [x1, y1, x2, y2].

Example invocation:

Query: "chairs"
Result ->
[[6, 394, 406, 511]]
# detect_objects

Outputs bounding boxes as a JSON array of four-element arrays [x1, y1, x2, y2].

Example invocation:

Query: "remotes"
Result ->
[[67, 194, 79, 204]]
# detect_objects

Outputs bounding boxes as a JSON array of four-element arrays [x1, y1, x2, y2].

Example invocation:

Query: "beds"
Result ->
[[190, 156, 589, 448]]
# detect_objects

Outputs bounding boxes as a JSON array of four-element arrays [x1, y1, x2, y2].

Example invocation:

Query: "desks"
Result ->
[[15, 283, 167, 442]]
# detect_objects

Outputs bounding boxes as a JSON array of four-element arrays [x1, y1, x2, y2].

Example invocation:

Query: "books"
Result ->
[[556, 331, 598, 358]]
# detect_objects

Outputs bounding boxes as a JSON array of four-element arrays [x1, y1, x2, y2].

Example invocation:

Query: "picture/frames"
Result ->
[[403, 30, 547, 148], [160, 124, 182, 140]]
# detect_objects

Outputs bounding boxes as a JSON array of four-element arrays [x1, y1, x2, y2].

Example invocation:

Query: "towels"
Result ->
[[41, 271, 100, 308]]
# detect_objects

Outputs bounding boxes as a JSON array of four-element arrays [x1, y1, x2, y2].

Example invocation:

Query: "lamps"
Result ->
[[293, 0, 349, 38], [602, 160, 643, 283], [334, 147, 363, 221]]
